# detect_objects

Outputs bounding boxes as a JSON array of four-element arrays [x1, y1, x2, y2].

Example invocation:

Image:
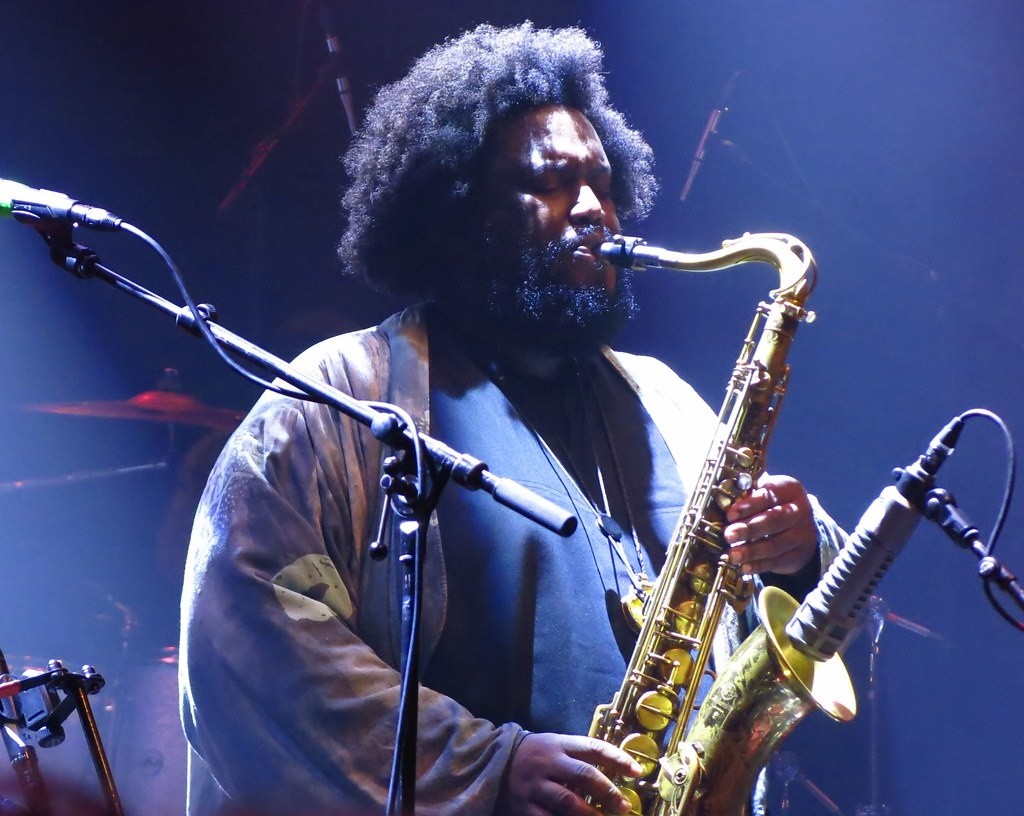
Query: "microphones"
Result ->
[[0, 179, 124, 230], [865, 594, 934, 637], [782, 772, 790, 810], [315, 0, 359, 136], [0, 650, 50, 816], [785, 417, 967, 662], [680, 72, 741, 202]]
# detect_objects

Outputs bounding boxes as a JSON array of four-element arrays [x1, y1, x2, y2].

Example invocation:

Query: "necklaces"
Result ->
[[480, 351, 655, 636]]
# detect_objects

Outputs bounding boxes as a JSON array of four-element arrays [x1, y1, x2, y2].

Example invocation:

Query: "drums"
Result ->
[[0, 654, 120, 793], [113, 644, 190, 816]]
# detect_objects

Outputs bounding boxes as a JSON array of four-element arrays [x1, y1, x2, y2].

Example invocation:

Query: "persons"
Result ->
[[178, 20, 852, 816]]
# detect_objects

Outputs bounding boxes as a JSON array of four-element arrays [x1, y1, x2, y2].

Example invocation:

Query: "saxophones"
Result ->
[[578, 227, 857, 816]]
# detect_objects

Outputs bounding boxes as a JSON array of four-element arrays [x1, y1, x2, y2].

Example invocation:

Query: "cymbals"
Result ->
[[8, 388, 249, 434]]
